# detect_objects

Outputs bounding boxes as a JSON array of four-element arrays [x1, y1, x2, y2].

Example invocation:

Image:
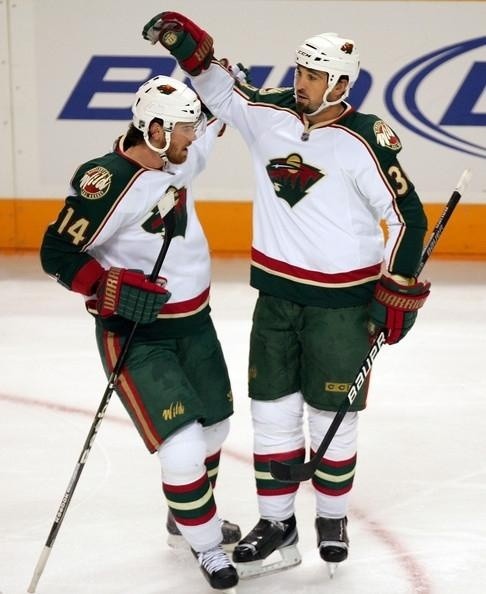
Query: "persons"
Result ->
[[141, 8, 432, 570], [37, 56, 263, 593]]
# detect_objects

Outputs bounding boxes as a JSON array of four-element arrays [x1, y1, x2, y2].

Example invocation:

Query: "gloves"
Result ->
[[367, 270, 432, 345], [95, 268, 173, 325], [140, 10, 214, 78], [196, 58, 252, 124]]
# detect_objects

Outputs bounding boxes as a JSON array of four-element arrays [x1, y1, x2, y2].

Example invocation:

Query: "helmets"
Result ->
[[292, 32, 362, 114], [131, 73, 207, 153]]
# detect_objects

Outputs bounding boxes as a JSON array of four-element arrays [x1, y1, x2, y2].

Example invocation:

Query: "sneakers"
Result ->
[[191, 544, 239, 590], [314, 517, 350, 563], [166, 504, 242, 544], [231, 514, 300, 563]]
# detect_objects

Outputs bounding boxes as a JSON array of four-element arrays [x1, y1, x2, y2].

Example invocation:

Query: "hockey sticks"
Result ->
[[28, 189, 176, 594], [268, 169, 474, 481]]
